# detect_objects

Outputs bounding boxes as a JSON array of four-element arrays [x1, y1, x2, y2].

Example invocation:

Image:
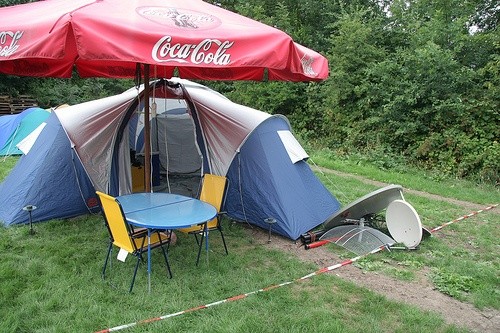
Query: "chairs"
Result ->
[[96, 190, 173, 293], [168, 174, 231, 267]]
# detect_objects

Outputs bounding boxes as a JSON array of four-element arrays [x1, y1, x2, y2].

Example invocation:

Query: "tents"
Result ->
[[0, 76, 344, 240]]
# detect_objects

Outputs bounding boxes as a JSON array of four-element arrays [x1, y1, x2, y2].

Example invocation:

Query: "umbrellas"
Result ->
[[0, 0, 328, 193]]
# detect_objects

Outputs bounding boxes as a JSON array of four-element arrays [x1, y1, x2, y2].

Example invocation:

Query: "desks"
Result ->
[[115, 193, 217, 290]]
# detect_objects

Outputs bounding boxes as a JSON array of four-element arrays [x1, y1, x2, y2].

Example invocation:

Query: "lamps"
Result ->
[[24, 205, 37, 212]]
